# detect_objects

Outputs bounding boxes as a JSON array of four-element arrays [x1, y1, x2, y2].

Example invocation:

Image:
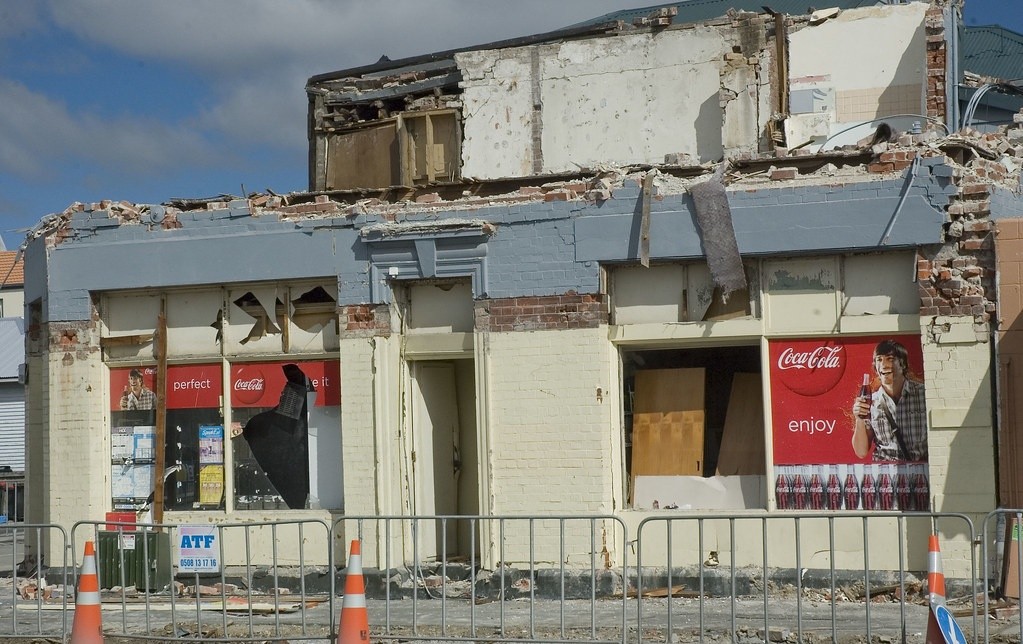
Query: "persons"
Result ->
[[852, 340, 928, 462], [119, 369, 157, 410]]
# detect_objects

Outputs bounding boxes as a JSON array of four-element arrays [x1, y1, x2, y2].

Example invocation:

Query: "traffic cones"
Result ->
[[337, 540, 372, 644], [926, 534, 946, 644], [70, 540, 106, 644]]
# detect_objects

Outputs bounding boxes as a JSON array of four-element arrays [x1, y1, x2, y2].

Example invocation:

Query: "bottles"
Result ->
[[121, 385, 128, 408], [774, 464, 930, 512], [859, 373, 872, 419]]
